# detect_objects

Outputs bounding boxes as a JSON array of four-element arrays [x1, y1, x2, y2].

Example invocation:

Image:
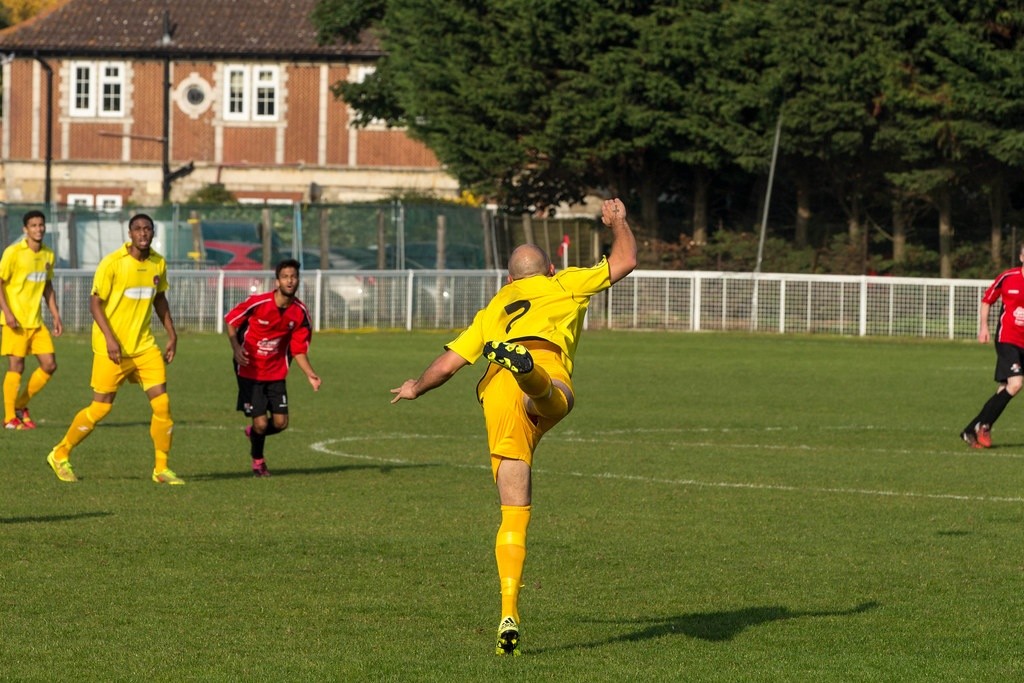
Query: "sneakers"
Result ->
[[15, 406, 36, 429], [482, 340, 533, 375], [251, 458, 270, 477], [245, 426, 251, 437], [958, 432, 985, 448], [3, 417, 30, 430], [496, 618, 521, 658], [46, 450, 79, 482], [152, 467, 185, 485], [974, 422, 991, 447]]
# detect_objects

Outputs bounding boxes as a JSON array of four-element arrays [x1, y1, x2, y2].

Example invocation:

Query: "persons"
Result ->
[[0, 211, 63, 430], [222, 260, 322, 478], [47, 214, 187, 485], [391, 198, 637, 656], [959, 244, 1024, 448]]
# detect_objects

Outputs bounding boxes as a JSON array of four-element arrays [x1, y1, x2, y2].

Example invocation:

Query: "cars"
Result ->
[[280, 246, 451, 321]]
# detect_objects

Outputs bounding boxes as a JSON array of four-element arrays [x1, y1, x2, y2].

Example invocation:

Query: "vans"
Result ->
[[10, 221, 280, 319], [392, 243, 481, 269]]
